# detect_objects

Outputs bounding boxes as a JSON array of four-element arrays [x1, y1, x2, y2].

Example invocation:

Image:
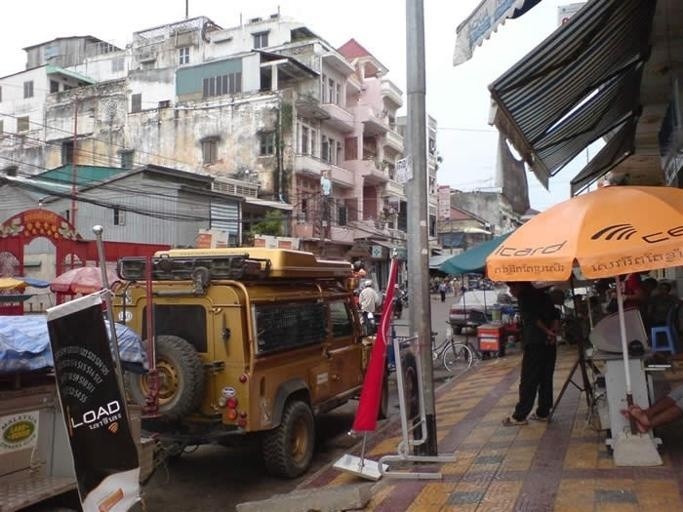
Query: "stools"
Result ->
[[651, 325, 678, 356]]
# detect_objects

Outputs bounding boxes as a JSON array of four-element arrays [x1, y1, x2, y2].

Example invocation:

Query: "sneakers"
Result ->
[[501, 415, 528, 426], [527, 411, 553, 422]]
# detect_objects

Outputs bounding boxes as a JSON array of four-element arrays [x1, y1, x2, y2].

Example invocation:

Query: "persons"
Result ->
[[437, 281, 446, 301], [612, 273, 642, 308], [395, 284, 405, 319], [449, 277, 457, 298], [502, 281, 561, 425], [621, 381, 682, 433], [644, 278, 658, 305], [358, 280, 380, 325], [642, 283, 682, 332]]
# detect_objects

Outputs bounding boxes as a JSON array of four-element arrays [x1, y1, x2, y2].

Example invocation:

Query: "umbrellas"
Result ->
[[50, 267, 119, 296], [484, 185, 682, 431], [0, 278, 49, 294]]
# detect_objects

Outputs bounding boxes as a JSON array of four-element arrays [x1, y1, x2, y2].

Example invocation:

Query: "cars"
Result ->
[[447, 288, 512, 335]]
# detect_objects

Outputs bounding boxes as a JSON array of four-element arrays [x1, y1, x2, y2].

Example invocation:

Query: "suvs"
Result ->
[[100, 243, 392, 482]]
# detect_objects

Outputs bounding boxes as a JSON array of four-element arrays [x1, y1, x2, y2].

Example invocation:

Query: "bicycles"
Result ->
[[428, 321, 473, 373]]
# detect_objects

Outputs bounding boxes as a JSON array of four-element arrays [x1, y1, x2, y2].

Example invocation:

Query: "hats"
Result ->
[[394, 283, 400, 289], [364, 281, 372, 286]]
